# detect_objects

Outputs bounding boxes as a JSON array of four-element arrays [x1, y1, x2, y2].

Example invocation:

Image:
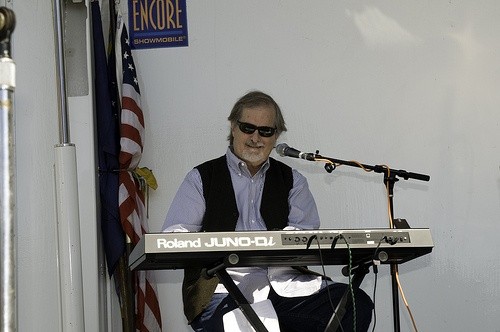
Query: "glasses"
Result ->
[[234, 120, 276, 137]]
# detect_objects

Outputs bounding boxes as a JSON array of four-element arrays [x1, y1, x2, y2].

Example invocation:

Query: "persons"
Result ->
[[159, 92, 373, 332]]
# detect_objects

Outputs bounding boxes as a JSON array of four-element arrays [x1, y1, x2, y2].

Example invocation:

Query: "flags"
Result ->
[[90, 0, 163, 332]]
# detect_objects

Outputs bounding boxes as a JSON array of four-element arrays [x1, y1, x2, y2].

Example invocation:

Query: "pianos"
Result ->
[[128, 228, 433, 272]]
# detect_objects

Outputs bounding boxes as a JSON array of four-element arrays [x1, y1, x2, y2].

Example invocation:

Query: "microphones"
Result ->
[[276, 142, 315, 162]]
